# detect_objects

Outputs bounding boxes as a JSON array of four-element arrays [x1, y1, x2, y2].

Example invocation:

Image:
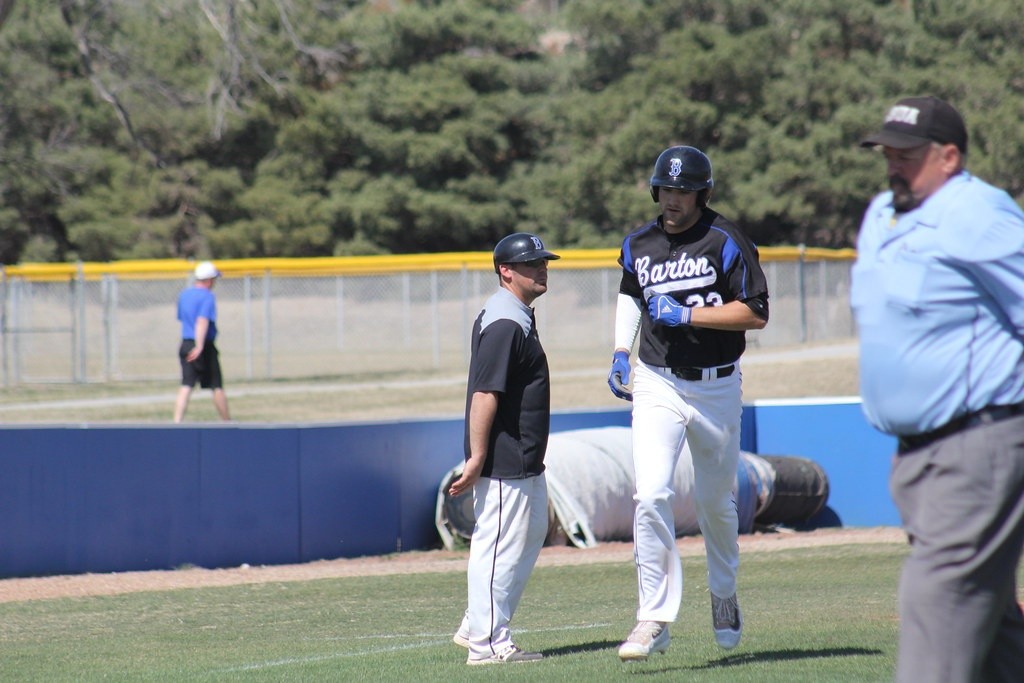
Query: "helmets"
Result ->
[[650, 145, 713, 202]]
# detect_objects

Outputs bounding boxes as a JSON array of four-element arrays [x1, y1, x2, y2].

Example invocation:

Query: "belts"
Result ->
[[896, 401, 1023, 454], [672, 365, 735, 381]]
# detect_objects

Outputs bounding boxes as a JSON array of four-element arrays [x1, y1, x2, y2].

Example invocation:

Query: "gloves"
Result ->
[[607, 351, 633, 402], [647, 290, 692, 327]]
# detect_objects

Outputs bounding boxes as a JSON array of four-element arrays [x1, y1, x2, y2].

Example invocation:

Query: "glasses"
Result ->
[[522, 258, 549, 268]]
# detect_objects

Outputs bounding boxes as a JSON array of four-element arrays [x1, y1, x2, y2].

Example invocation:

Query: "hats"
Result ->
[[194, 262, 222, 280], [860, 95, 968, 153], [493, 233, 561, 274]]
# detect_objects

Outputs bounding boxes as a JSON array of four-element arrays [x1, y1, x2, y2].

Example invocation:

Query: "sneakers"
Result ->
[[453, 620, 470, 648], [465, 645, 544, 664], [617, 621, 671, 662], [711, 591, 744, 650]]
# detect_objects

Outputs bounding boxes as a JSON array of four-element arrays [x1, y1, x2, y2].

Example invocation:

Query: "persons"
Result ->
[[448, 232, 561, 665], [172, 261, 232, 424], [604, 145, 769, 662], [846, 94, 1023, 682]]
